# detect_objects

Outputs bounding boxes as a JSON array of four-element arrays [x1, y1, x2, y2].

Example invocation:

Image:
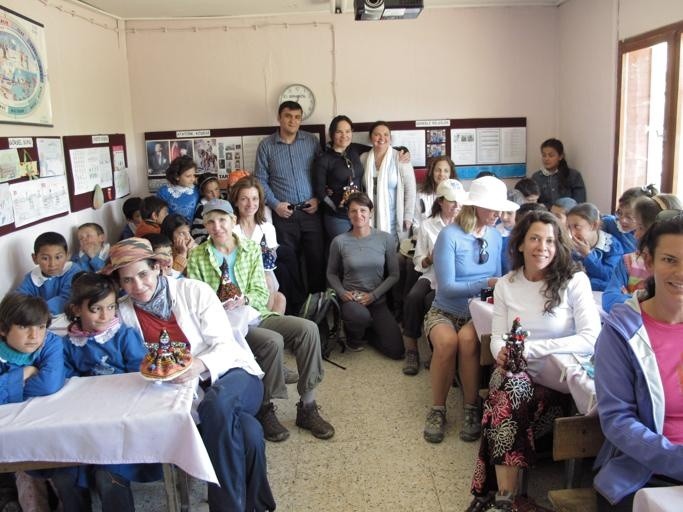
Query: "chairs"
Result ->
[[542, 417, 606, 512], [473, 334, 494, 405]]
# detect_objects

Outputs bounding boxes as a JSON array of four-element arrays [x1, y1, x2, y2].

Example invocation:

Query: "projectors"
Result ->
[[354, 0, 424, 20]]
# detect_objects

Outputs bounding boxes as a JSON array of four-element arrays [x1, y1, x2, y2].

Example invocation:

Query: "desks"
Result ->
[[545, 351, 599, 413], [46, 304, 250, 344], [0, 369, 222, 511], [460, 287, 605, 334], [629, 484, 682, 512]]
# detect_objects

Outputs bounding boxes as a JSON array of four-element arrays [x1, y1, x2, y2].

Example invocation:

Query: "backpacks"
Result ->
[[298, 287, 347, 371]]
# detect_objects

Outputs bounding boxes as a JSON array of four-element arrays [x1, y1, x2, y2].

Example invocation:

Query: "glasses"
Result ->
[[475, 237, 489, 265], [656, 208, 683, 222]]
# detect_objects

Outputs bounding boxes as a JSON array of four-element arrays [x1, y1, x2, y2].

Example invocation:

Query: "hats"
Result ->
[[101, 237, 174, 276], [437, 179, 521, 212], [201, 198, 233, 218], [228, 168, 251, 188]]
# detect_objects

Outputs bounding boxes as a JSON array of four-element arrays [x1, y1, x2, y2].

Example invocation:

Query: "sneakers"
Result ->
[[342, 322, 364, 353], [423, 408, 447, 442], [254, 402, 289, 442], [402, 349, 419, 374], [460, 395, 484, 442], [296, 398, 334, 439]]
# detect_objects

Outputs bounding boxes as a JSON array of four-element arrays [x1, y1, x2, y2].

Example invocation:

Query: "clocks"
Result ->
[[277, 83, 317, 122]]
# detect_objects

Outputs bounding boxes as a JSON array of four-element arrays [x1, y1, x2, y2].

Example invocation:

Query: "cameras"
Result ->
[[353, 291, 365, 301]]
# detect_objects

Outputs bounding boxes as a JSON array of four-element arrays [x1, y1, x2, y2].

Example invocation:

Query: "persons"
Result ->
[[255, 100, 418, 361], [403, 138, 682, 512], [1, 153, 335, 512]]
